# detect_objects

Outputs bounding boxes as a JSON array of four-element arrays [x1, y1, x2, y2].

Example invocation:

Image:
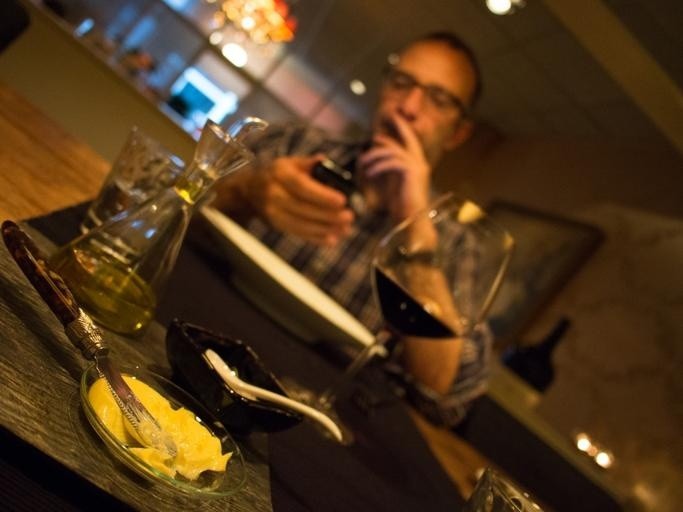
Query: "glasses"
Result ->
[[383, 68, 471, 119]]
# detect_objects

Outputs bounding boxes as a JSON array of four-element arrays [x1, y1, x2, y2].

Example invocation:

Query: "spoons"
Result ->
[[205, 345, 343, 444]]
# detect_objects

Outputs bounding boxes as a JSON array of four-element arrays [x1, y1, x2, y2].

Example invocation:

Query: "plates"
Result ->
[[80, 361, 247, 501], [175, 195, 388, 362]]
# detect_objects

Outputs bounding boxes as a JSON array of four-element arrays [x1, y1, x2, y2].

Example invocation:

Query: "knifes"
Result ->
[[1, 219, 180, 462]]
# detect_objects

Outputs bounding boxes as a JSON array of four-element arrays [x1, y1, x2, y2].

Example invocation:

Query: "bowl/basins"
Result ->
[[164, 315, 300, 438]]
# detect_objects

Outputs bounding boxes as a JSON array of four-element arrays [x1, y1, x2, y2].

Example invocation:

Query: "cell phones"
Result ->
[[311, 156, 356, 199]]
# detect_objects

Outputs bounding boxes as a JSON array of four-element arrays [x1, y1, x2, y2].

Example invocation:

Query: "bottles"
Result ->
[[45, 120, 253, 337], [493, 311, 576, 409]]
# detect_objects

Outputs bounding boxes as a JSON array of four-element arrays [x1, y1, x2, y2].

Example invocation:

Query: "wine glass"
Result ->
[[274, 188, 519, 446]]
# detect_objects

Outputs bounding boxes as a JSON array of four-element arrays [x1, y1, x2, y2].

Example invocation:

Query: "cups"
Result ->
[[75, 124, 219, 275], [461, 469, 545, 511]]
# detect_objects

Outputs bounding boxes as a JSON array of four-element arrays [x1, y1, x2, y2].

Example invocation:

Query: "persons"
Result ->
[[168, 23, 499, 431]]
